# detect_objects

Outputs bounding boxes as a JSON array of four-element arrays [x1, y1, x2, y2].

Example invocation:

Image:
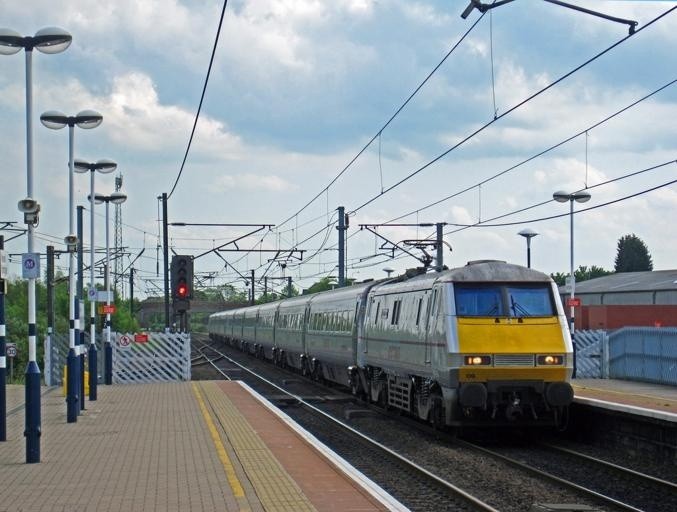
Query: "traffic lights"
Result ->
[[170, 255, 194, 301]]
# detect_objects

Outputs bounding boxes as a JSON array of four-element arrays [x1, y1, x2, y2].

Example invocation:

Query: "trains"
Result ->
[[209, 260, 574, 449]]
